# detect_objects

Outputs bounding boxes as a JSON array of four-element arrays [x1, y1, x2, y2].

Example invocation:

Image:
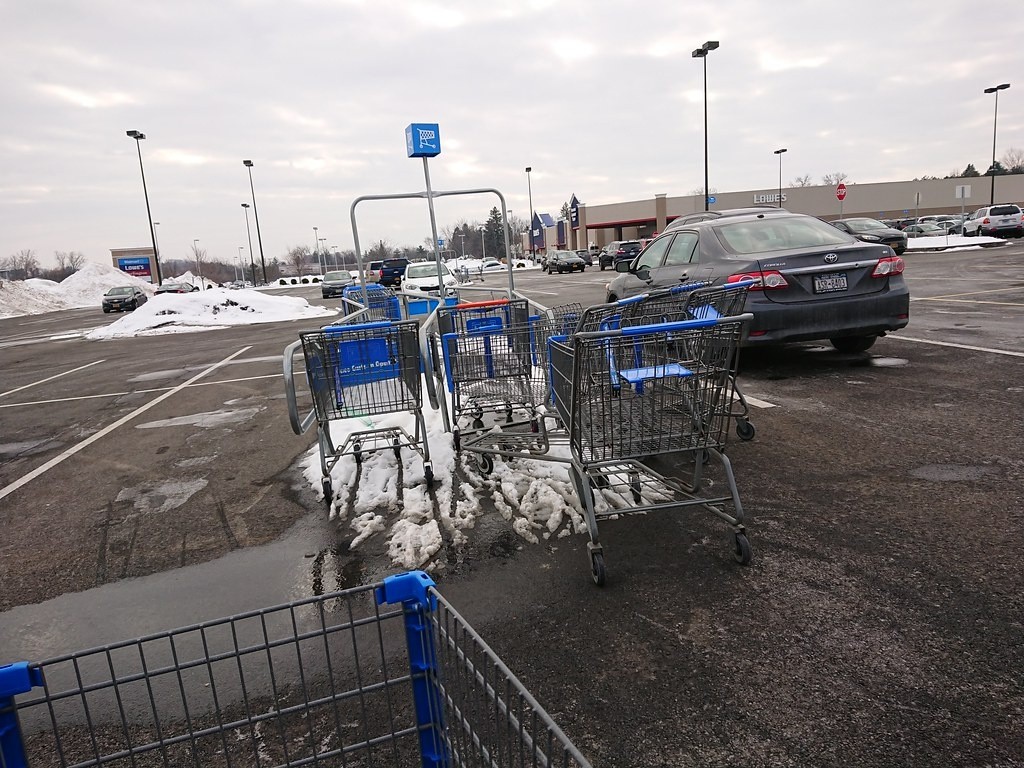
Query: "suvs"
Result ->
[[962, 201, 1024, 239], [599, 205, 788, 271]]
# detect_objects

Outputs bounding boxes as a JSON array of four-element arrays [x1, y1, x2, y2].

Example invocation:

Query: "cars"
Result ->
[[101, 282, 200, 313], [527, 248, 594, 275], [827, 214, 969, 256], [478, 258, 508, 272], [605, 212, 909, 365], [322, 259, 459, 299]]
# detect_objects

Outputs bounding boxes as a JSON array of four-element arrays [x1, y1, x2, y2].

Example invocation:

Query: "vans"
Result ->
[[588, 246, 600, 257]]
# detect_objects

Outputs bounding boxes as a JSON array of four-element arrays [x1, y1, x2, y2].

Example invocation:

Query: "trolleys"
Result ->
[[0, 570, 592, 768], [300, 280, 758, 585]]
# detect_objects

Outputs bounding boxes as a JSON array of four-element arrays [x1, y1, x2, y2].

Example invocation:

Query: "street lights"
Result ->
[[126, 130, 164, 286], [233, 160, 268, 283], [194, 239, 200, 276], [508, 167, 535, 260], [692, 41, 719, 213], [984, 83, 1010, 205], [313, 227, 339, 275], [774, 149, 787, 208]]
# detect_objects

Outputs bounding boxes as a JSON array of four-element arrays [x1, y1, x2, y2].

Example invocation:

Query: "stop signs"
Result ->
[[836, 184, 846, 200]]
